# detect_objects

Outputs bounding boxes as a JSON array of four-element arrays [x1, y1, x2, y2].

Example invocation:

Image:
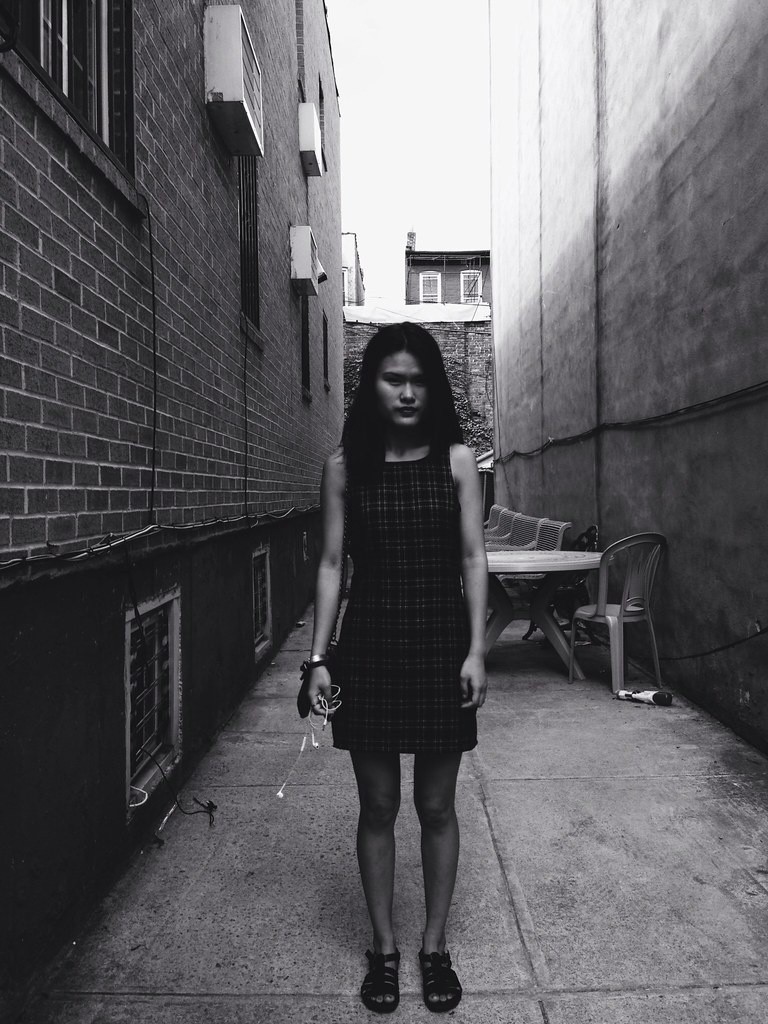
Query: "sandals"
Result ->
[[360, 949, 402, 1013], [417, 945, 463, 1013]]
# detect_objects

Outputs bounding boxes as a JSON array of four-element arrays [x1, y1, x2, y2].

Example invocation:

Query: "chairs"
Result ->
[[483, 504, 573, 616], [569, 531, 667, 694]]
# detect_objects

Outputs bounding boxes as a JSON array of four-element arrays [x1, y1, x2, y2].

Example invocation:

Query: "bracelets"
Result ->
[[308, 654, 326, 663]]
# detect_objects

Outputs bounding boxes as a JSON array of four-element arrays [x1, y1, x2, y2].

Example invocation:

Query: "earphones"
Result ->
[[276, 784, 285, 799], [311, 733, 319, 750]]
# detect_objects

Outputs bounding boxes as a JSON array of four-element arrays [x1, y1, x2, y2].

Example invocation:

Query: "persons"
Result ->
[[296, 322, 489, 1016]]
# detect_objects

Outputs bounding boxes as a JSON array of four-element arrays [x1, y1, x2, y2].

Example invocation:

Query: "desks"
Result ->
[[483, 550, 617, 681]]
